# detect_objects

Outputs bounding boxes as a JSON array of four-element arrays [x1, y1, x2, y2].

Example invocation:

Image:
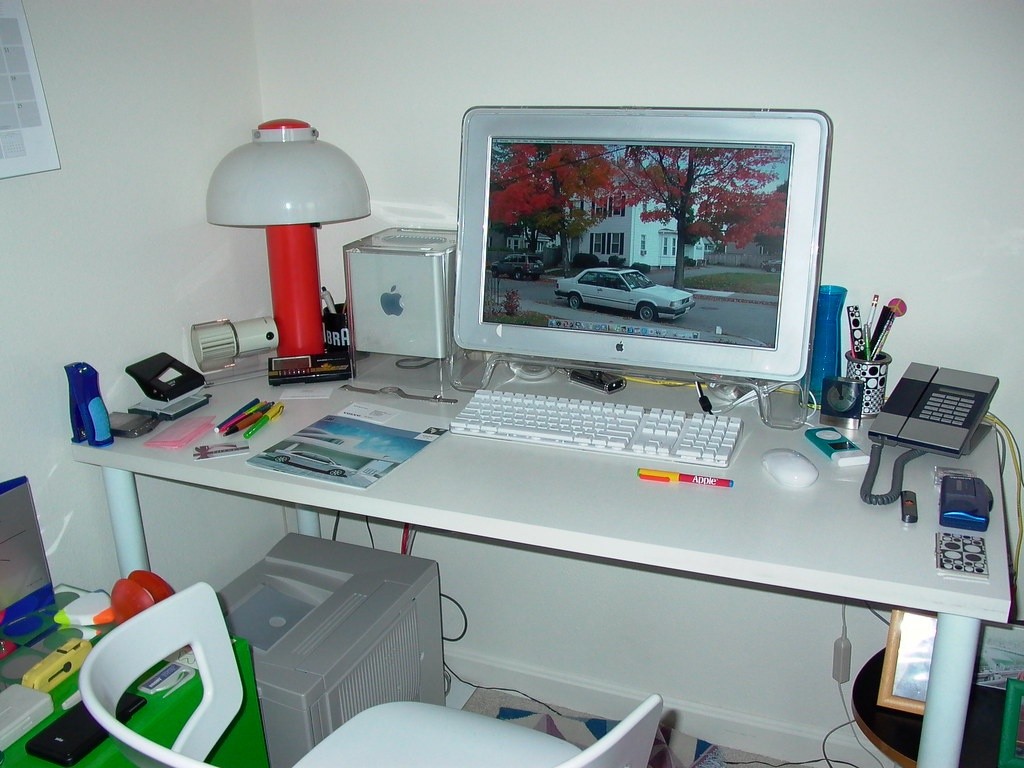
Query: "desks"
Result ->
[[851, 648, 1005, 768], [72, 348, 1012, 768], [0, 583, 269, 768]]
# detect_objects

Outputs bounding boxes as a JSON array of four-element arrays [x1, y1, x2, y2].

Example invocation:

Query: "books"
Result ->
[[247, 401, 452, 489]]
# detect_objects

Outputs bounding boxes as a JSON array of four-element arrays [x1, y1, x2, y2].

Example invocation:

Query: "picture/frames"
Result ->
[[877, 608, 938, 715], [998, 678, 1024, 768]]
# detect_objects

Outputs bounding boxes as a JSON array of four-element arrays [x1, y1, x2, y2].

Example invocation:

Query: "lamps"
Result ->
[[205, 118, 372, 356]]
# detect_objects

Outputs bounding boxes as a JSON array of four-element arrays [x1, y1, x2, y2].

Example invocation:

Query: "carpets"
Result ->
[[458, 685, 809, 768]]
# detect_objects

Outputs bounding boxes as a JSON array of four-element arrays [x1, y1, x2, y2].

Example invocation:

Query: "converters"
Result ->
[[832, 637, 851, 684]]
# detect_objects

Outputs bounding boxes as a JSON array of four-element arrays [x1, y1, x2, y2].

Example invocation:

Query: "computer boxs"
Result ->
[[210, 530, 445, 768]]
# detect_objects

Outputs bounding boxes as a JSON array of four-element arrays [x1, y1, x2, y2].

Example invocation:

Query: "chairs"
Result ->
[[77, 583, 664, 768]]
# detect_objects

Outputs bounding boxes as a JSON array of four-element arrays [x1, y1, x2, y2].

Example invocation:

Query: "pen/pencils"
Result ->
[[638, 468, 734, 487], [863, 295, 897, 361], [214, 397, 287, 438]]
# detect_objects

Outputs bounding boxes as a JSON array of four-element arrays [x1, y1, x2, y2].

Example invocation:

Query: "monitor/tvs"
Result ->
[[451, 107, 833, 428]]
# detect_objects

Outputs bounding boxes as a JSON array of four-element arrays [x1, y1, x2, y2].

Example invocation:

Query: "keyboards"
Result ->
[[450, 389, 745, 467]]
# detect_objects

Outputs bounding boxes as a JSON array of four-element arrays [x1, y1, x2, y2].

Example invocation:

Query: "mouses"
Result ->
[[761, 449, 819, 487]]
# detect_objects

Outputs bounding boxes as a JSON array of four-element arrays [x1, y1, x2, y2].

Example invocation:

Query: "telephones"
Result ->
[[867, 361, 1002, 457]]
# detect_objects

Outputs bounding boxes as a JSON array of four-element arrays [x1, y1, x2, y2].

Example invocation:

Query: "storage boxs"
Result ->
[[342, 227, 458, 360]]
